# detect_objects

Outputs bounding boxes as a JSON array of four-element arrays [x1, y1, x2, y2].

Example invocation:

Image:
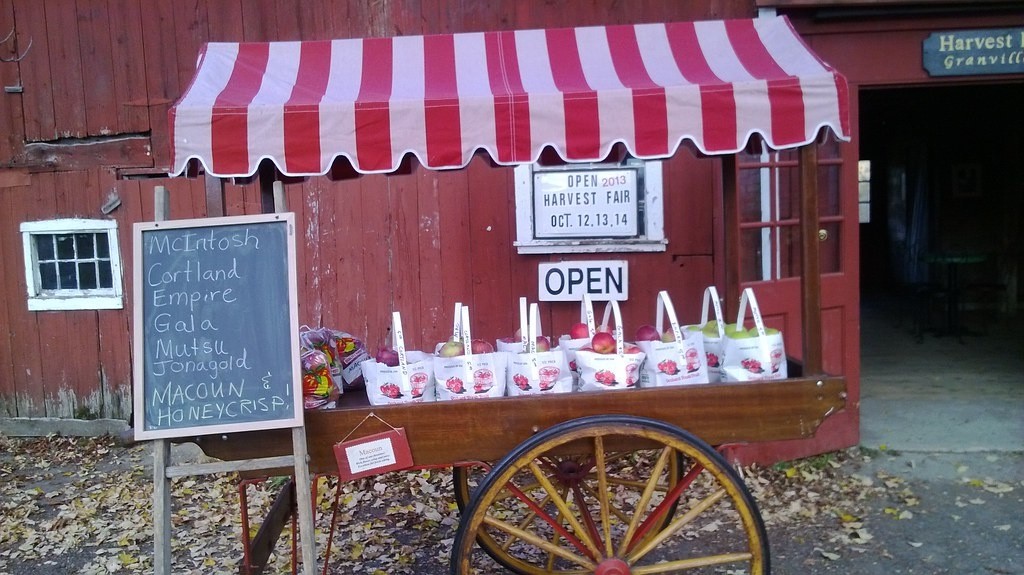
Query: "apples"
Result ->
[[299, 337, 373, 408], [438, 338, 494, 357], [688, 320, 779, 338], [502, 321, 685, 354], [376, 346, 399, 367]]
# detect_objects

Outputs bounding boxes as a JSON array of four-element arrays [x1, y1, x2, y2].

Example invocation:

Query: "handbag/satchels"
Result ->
[[506, 302, 572, 397], [433, 306, 506, 401], [682, 285, 724, 381], [496, 297, 551, 352], [718, 287, 788, 382], [635, 291, 709, 388], [362, 313, 433, 406], [558, 294, 618, 392], [434, 302, 462, 355], [574, 299, 647, 392]]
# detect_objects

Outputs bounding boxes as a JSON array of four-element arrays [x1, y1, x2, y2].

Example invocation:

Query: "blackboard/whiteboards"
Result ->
[[129, 211, 306, 444]]
